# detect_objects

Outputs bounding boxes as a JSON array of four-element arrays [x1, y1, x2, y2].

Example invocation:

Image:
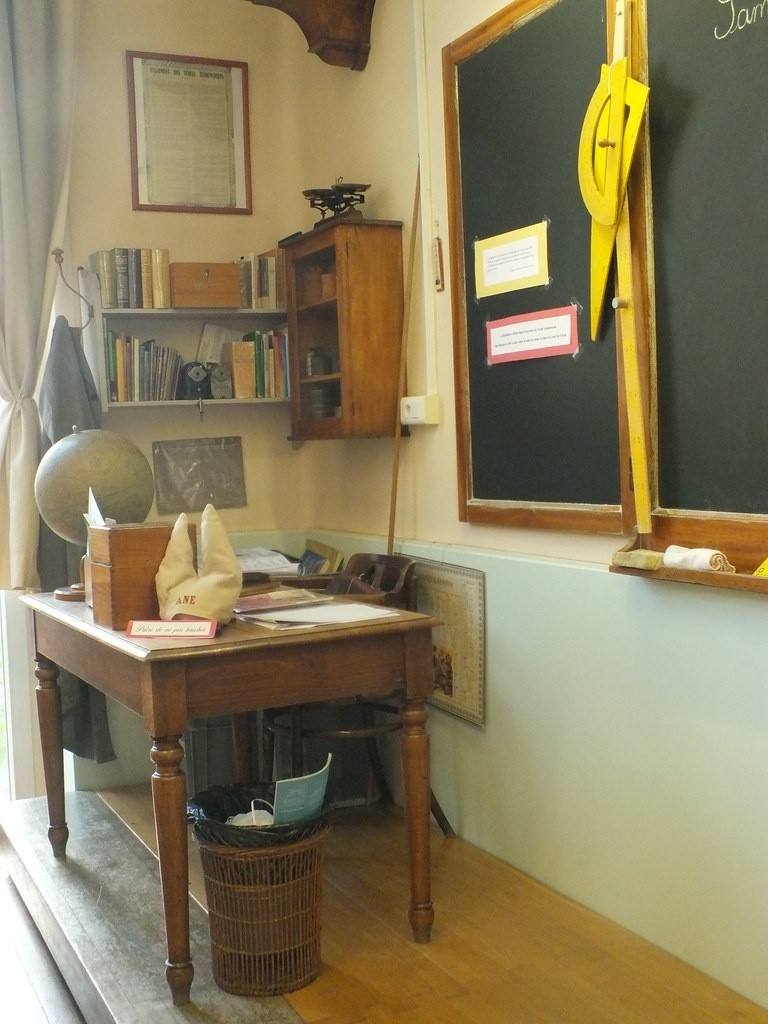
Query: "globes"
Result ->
[[33, 425, 156, 602]]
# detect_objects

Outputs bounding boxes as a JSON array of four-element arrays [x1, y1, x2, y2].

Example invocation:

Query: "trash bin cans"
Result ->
[[188, 784, 334, 999]]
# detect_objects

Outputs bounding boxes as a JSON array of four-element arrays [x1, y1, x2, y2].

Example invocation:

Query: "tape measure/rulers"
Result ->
[[577, 57, 651, 342]]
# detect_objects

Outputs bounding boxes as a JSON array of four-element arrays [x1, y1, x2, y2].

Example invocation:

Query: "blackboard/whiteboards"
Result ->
[[437, 0, 766, 590]]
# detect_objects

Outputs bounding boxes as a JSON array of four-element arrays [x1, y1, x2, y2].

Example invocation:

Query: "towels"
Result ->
[[662, 543, 737, 574]]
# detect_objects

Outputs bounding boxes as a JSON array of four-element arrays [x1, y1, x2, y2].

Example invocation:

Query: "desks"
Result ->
[[17, 585, 445, 1009]]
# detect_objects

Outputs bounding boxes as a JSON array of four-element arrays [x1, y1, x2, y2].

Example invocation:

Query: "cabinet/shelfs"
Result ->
[[96, 307, 289, 412], [277, 217, 411, 441]]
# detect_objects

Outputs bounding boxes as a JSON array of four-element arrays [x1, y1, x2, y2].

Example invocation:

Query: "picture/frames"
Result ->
[[125, 49, 253, 215]]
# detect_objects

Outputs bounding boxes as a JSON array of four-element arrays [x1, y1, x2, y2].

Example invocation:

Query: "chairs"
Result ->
[[259, 553, 458, 839], [188, 539, 345, 796]]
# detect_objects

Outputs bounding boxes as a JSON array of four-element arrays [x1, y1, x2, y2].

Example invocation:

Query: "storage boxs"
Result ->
[[169, 262, 241, 309]]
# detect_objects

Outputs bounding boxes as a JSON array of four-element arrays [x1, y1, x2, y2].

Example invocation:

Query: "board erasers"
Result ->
[[610, 547, 665, 571]]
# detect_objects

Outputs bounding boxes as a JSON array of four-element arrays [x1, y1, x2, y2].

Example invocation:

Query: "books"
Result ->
[[233, 588, 335, 614], [195, 322, 291, 399], [99, 247, 172, 309], [233, 253, 278, 309], [273, 752, 332, 824], [298, 538, 344, 575], [102, 317, 183, 402]]
